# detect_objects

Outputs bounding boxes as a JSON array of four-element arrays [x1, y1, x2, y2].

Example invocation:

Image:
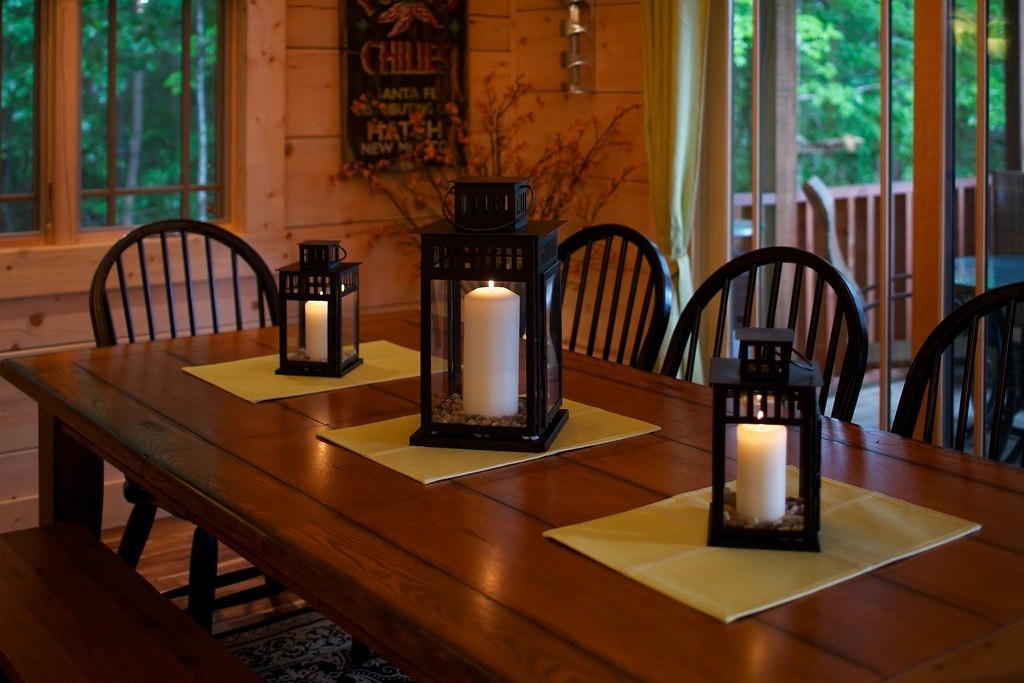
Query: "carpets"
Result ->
[[161, 567, 421, 683]]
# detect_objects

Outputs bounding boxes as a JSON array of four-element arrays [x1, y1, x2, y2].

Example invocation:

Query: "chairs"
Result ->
[[802, 175, 968, 370], [662, 246, 869, 422], [90, 220, 280, 595], [891, 281, 1024, 468], [556, 224, 673, 373]]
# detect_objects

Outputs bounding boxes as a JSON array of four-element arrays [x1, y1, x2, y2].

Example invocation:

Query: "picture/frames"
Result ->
[[339, 0, 469, 178]]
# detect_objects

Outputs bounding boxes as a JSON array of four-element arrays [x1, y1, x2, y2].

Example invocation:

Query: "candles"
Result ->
[[304, 291, 328, 363], [458, 282, 520, 418]]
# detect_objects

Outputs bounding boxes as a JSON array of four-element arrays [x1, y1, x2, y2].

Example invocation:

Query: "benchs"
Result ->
[[0, 522, 258, 683]]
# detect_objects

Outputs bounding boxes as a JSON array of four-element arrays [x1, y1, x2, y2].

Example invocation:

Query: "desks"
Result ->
[[953, 255, 1024, 439], [0, 303, 1024, 683]]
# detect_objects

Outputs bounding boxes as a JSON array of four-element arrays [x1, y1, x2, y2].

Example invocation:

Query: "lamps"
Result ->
[[734, 413, 788, 525]]
